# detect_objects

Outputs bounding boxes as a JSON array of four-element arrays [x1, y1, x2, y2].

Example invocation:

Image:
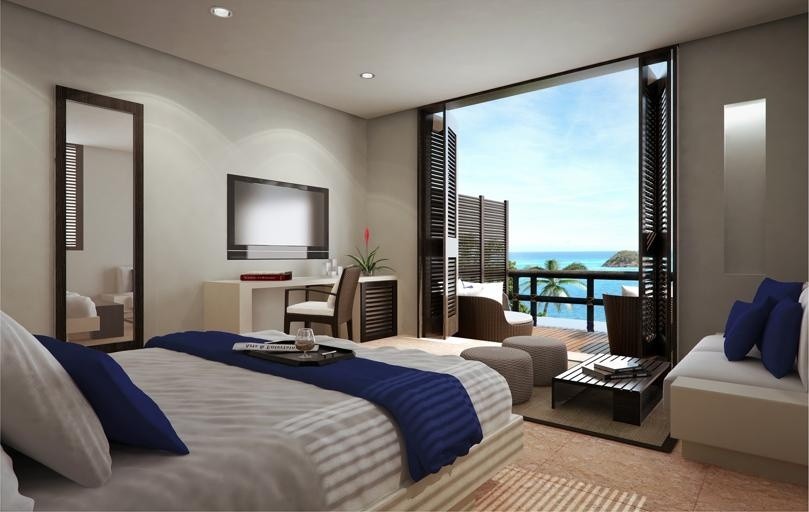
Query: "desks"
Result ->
[[202, 273, 400, 345]]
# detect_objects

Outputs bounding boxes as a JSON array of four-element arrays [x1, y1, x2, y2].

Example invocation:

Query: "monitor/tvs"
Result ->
[[226, 173, 330, 260]]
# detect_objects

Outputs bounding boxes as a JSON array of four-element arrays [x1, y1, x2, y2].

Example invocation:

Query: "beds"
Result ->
[[0, 287, 529, 512], [65, 289, 102, 343]]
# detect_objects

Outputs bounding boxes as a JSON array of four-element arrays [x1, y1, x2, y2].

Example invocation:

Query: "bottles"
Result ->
[[327, 252, 337, 279]]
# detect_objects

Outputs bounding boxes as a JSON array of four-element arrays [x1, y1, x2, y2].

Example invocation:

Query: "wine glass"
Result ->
[[294, 328, 316, 358]]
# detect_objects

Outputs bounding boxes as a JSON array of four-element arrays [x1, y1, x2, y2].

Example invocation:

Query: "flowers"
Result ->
[[345, 224, 397, 276]]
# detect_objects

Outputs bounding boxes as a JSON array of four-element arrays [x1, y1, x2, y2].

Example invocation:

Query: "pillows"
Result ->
[[463, 280, 504, 306], [621, 284, 654, 322], [718, 278, 805, 383], [0, 306, 117, 495], [35, 331, 207, 461], [439, 276, 464, 295]]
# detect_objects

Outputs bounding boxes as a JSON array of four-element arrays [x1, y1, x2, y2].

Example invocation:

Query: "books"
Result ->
[[581, 360, 647, 381], [240, 271, 292, 280]]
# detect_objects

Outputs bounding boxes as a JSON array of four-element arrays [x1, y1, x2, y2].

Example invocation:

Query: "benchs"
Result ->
[[661, 279, 806, 490], [101, 264, 134, 312]]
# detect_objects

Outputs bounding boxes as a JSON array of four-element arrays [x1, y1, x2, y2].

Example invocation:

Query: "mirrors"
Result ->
[[54, 80, 147, 351]]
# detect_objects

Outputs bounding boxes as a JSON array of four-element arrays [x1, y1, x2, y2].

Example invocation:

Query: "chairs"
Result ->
[[282, 263, 362, 342], [602, 287, 677, 360], [430, 291, 534, 343]]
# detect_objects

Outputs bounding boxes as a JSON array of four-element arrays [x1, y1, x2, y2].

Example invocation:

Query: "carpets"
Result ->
[[510, 357, 680, 456]]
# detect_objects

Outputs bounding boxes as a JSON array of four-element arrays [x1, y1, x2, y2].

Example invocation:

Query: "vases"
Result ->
[[363, 269, 374, 276]]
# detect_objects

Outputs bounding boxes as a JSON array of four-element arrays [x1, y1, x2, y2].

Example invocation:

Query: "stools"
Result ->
[[91, 298, 126, 341], [502, 335, 569, 389], [459, 345, 535, 406]]
